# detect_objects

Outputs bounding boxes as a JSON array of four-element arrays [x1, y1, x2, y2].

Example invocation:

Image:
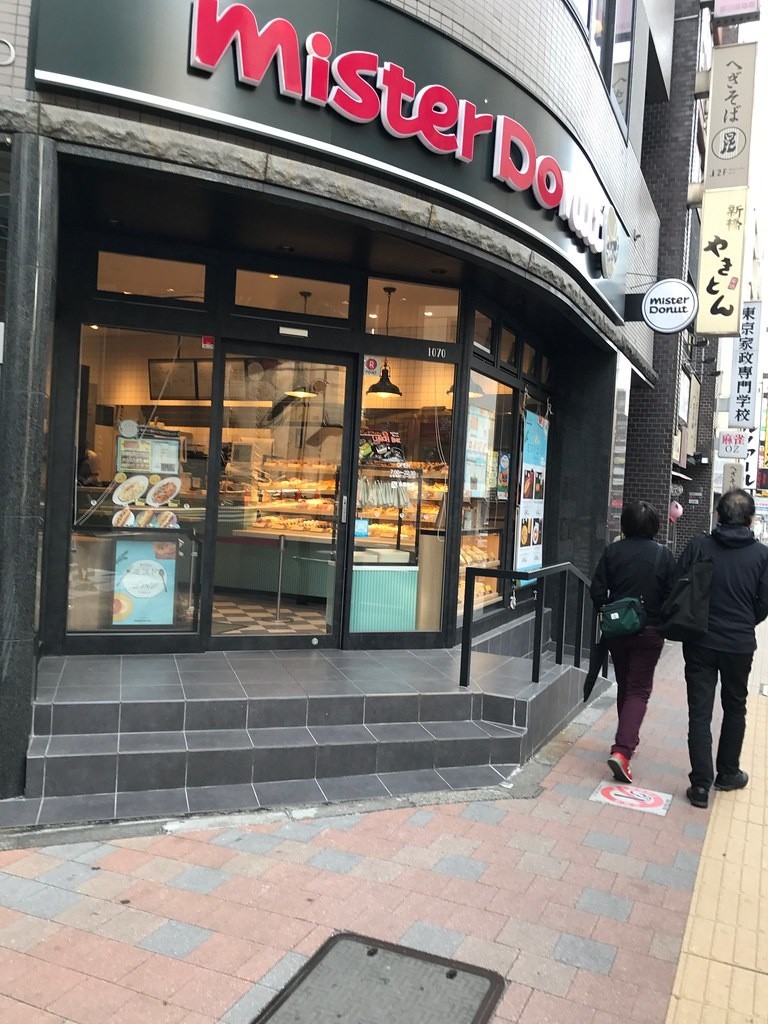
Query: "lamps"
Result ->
[[688, 356, 718, 365], [449, 378, 484, 404], [698, 370, 724, 378], [684, 337, 710, 350], [284, 292, 321, 397], [364, 285, 401, 397]]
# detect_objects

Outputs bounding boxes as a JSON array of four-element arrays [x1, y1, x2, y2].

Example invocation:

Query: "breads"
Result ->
[[254, 458, 489, 565]]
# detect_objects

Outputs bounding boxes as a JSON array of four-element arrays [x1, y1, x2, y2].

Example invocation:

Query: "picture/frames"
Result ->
[[109, 537, 179, 629]]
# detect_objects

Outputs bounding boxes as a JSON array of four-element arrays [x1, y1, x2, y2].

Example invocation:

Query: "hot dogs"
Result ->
[[136, 510, 153, 527], [114, 508, 130, 527], [157, 511, 173, 528]]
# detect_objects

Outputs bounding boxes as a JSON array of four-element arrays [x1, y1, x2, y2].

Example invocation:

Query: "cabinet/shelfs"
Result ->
[[420, 525, 505, 628], [253, 453, 449, 549]]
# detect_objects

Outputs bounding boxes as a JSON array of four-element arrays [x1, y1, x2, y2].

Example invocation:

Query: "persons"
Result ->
[[590, 501, 678, 782], [671, 487, 768, 809]]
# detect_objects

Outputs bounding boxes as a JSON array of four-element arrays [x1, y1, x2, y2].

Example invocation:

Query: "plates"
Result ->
[[124, 560, 167, 598], [112, 475, 149, 505], [112, 510, 177, 527], [146, 476, 181, 506], [113, 593, 131, 622], [248, 362, 265, 381]]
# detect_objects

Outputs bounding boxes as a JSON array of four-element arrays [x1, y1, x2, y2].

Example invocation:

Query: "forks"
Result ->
[[116, 565, 131, 586]]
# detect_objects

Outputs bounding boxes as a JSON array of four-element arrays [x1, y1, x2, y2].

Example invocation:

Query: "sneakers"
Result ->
[[686, 771, 749, 808], [606, 752, 635, 783]]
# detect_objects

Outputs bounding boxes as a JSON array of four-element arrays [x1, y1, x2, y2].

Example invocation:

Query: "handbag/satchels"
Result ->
[[655, 534, 713, 643], [598, 597, 644, 638]]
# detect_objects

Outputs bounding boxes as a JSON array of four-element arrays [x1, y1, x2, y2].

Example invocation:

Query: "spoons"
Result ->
[[159, 569, 167, 592]]
